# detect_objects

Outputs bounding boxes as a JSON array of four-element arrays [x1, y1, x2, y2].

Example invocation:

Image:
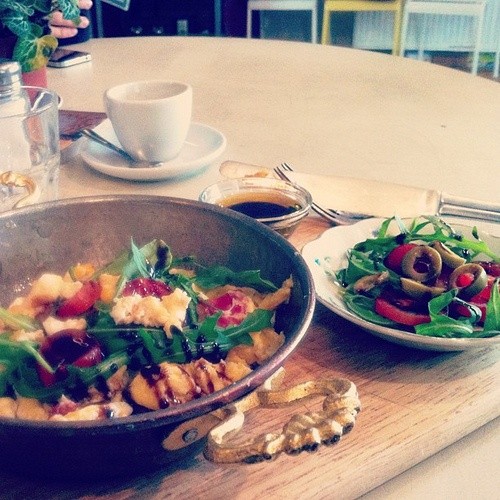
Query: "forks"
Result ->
[[274, 162, 372, 225]]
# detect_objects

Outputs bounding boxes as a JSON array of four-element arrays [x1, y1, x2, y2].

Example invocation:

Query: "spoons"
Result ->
[[80, 127, 163, 168]]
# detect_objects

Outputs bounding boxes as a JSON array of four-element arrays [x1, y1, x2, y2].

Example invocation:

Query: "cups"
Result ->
[[104, 80, 192, 162], [0, 87, 62, 210]]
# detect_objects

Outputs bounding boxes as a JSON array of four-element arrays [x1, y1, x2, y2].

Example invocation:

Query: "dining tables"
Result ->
[[0, 36, 500, 499]]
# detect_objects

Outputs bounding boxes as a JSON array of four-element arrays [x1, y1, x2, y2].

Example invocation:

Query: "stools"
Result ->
[[247, 0, 485, 74]]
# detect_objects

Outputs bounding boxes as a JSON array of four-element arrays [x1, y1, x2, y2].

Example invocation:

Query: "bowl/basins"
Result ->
[[197, 177, 312, 241], [0, 194, 316, 437]]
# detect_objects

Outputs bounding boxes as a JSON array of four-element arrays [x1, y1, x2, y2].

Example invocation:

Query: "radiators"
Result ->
[[353, 0, 500, 54]]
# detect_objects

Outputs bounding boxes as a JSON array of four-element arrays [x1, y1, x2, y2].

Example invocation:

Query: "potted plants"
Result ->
[[0, 0, 81, 104]]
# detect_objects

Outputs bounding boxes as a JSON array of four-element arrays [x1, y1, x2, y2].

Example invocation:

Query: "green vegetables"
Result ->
[[0, 234, 280, 399], [335, 214, 500, 337]]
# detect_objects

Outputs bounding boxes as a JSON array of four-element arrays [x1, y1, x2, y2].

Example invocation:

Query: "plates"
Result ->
[[79, 119, 227, 181], [300, 218, 500, 352]]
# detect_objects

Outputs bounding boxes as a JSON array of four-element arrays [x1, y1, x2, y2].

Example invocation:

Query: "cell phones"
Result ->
[[47, 48, 91, 67]]
